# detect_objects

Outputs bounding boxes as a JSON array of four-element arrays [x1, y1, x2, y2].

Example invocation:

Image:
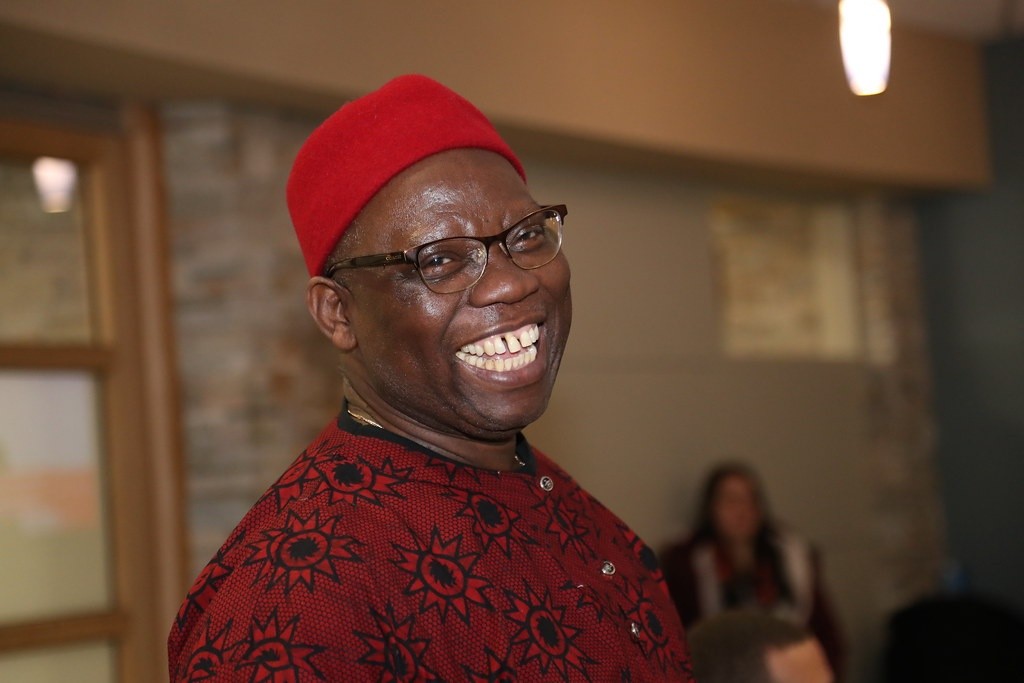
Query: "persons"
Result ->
[[683, 458, 837, 683], [164, 69, 699, 683]]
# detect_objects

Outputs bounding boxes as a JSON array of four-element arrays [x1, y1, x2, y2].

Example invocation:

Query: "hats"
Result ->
[[285, 74, 527, 280]]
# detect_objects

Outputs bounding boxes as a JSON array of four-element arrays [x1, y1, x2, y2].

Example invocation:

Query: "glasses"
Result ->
[[326, 205, 568, 295]]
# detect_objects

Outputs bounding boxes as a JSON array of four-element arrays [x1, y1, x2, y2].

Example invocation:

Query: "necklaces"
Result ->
[[347, 409, 525, 468]]
